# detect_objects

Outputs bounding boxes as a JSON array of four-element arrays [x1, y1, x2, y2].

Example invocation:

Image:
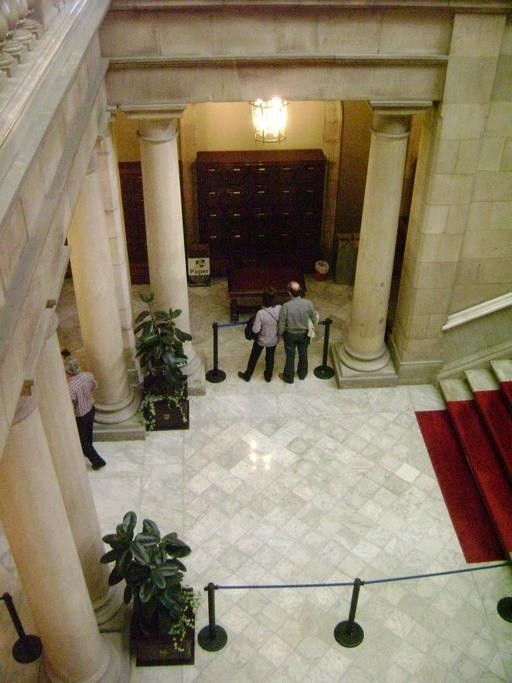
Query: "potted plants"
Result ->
[[135, 293, 193, 431], [100, 511, 202, 666]]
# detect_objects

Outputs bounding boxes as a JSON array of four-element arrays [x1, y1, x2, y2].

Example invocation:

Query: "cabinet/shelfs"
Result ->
[[197, 150, 327, 277]]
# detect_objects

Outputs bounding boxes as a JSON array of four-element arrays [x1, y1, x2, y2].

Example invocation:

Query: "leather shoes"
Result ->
[[279, 372, 293, 383], [238, 371, 250, 382], [92, 459, 106, 470], [264, 369, 271, 381]]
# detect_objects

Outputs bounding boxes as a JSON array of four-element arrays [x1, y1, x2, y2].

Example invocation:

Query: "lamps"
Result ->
[[250, 101, 288, 144]]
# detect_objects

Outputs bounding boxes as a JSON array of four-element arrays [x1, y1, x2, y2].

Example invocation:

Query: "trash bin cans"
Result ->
[[315, 260, 329, 281]]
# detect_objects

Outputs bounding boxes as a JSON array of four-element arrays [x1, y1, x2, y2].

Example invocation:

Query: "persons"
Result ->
[[64, 354, 107, 470], [278, 282, 318, 384], [238, 287, 282, 382]]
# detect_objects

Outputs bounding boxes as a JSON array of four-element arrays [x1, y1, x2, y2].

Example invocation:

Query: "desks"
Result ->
[[228, 265, 306, 321]]
